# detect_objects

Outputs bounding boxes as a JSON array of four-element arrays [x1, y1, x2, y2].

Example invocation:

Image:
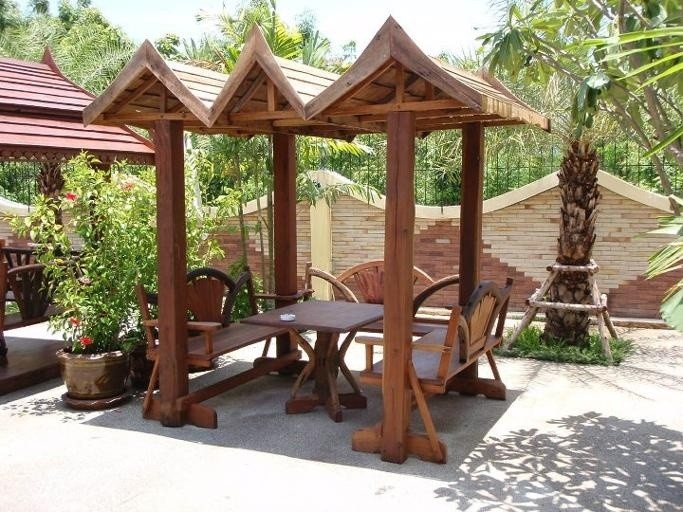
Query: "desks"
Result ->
[[239, 299, 384, 423]]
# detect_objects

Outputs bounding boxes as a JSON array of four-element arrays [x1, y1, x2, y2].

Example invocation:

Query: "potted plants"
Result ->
[[115, 160, 247, 389]]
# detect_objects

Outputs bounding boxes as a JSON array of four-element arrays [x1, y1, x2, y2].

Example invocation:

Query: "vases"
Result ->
[[55, 345, 132, 410]]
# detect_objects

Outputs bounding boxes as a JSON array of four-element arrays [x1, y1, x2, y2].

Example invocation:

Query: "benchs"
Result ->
[[130, 265, 313, 429], [356, 278, 513, 464], [304, 257, 460, 333], [1, 244, 81, 329]]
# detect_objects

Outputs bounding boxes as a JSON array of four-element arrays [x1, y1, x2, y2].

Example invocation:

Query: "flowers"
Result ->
[[0, 149, 158, 353]]
